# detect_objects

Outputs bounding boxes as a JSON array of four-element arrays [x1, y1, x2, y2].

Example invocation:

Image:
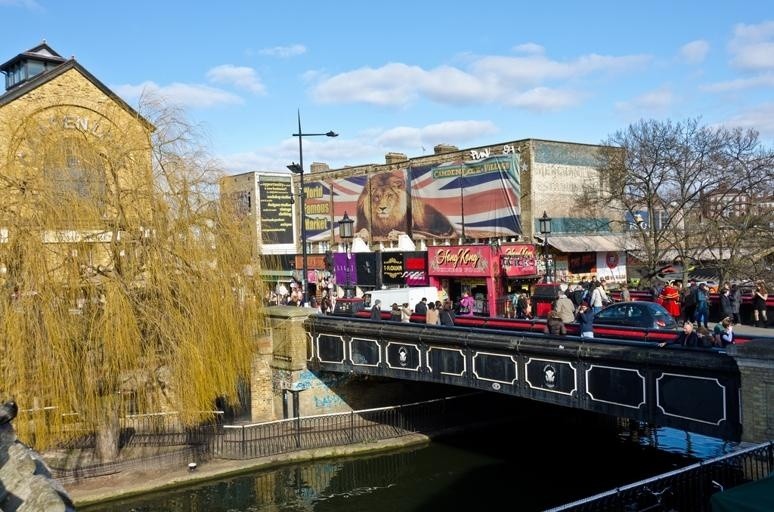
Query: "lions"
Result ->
[[351, 168, 475, 244]]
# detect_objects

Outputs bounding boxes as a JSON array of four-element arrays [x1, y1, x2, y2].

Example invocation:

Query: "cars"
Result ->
[[590, 300, 678, 331], [667, 279, 760, 293]]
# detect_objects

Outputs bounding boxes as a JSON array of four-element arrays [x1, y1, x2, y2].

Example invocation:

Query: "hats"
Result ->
[[581, 302, 588, 306], [375, 300, 380, 305]]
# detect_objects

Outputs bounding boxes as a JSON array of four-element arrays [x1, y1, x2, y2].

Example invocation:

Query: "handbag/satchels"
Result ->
[[459, 306, 470, 313]]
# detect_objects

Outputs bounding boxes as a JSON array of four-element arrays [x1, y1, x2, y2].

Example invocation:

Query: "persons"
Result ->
[[548, 276, 610, 338], [460, 290, 473, 316], [714, 282, 743, 344], [265, 288, 337, 314], [390, 297, 454, 325], [657, 278, 710, 347], [510, 290, 534, 320], [620, 285, 630, 302], [752, 282, 768, 328], [372, 299, 381, 320]]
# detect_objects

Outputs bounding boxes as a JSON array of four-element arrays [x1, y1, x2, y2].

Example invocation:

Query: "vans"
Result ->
[[362, 286, 439, 312]]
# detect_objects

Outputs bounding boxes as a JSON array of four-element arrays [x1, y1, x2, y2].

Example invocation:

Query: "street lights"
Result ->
[[338, 209, 356, 300], [287, 107, 342, 310], [538, 210, 554, 285]]
